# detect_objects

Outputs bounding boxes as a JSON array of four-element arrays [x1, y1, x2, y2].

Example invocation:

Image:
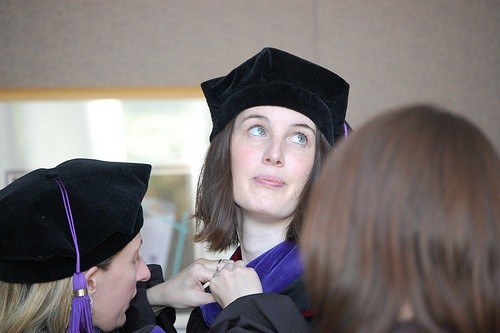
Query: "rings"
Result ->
[[216, 259, 222, 272]]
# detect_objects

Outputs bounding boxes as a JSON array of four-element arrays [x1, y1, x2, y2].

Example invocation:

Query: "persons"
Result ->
[[299, 105, 500, 333], [0, 157, 312, 333], [185, 47, 351, 333]]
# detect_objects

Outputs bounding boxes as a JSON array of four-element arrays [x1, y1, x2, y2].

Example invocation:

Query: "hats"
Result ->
[[200, 47, 350, 148], [0, 158, 152, 333]]
